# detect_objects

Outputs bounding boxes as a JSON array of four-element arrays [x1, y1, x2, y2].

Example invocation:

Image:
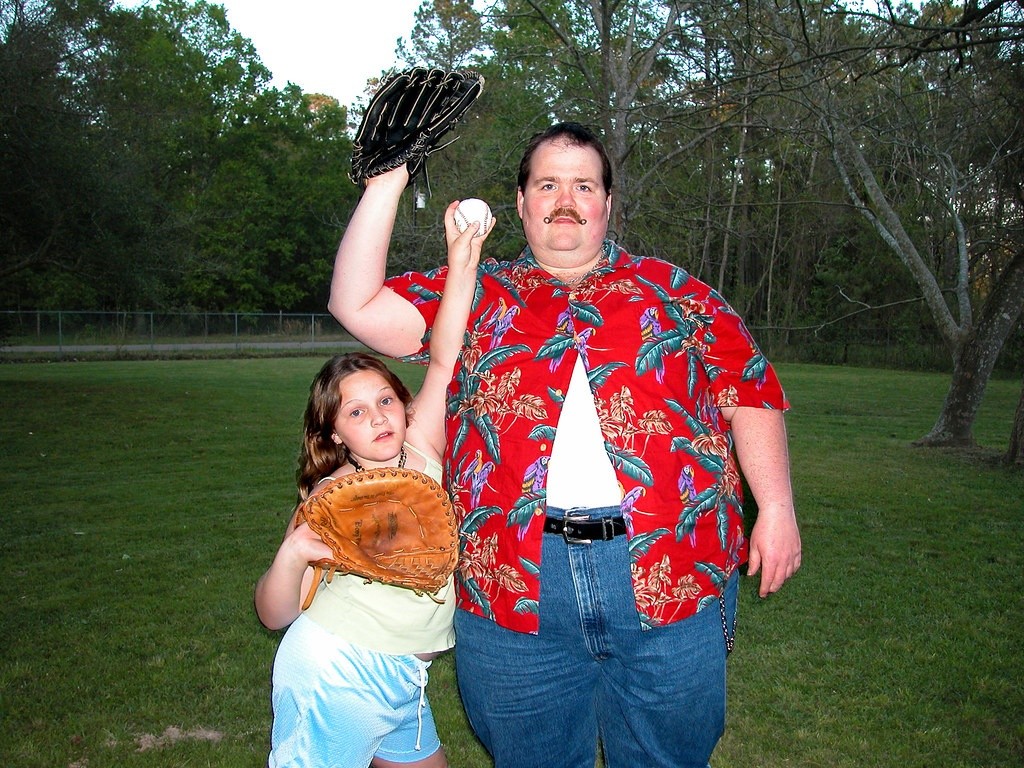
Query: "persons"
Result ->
[[327, 65, 803, 768], [257, 202, 502, 768]]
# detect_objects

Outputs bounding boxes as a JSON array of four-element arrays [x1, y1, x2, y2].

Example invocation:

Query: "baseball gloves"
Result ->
[[348, 66, 485, 199], [293, 466, 460, 613]]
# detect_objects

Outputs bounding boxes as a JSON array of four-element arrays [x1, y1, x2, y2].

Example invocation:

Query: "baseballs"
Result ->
[[454, 198, 492, 237]]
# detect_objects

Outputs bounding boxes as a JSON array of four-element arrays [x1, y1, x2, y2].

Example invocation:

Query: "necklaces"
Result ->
[[344, 445, 407, 472]]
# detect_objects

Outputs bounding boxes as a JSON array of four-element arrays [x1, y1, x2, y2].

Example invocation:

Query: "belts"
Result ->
[[541, 516, 633, 550]]
